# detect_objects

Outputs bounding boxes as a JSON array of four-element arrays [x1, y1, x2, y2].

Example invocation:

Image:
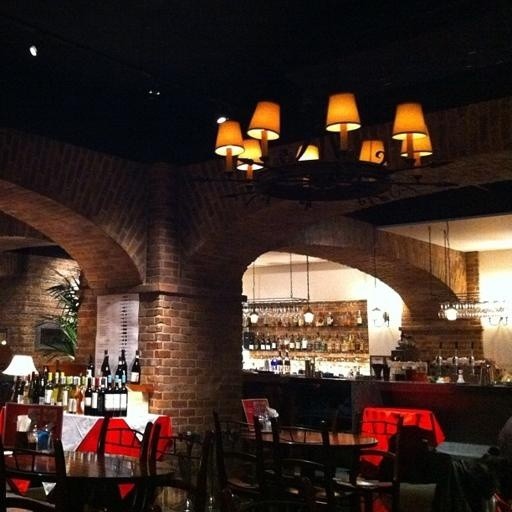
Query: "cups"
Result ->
[[371, 362, 384, 379]]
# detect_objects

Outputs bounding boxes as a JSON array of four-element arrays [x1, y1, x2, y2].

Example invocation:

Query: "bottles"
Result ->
[[243, 326, 369, 354], [84, 347, 142, 384], [270, 348, 291, 375], [248, 304, 365, 327], [9, 370, 129, 416]]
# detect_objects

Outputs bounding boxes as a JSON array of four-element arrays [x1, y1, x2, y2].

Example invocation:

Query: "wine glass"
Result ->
[[435, 300, 506, 321], [241, 300, 307, 325]]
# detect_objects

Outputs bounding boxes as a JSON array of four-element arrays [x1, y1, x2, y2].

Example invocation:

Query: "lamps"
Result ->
[[444, 221, 458, 321], [250, 263, 258, 324], [303, 254, 315, 323], [208, 80, 454, 206], [2, 353, 40, 381]]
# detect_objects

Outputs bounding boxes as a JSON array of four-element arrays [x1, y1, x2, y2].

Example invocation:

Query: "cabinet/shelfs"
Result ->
[[246, 298, 370, 363]]
